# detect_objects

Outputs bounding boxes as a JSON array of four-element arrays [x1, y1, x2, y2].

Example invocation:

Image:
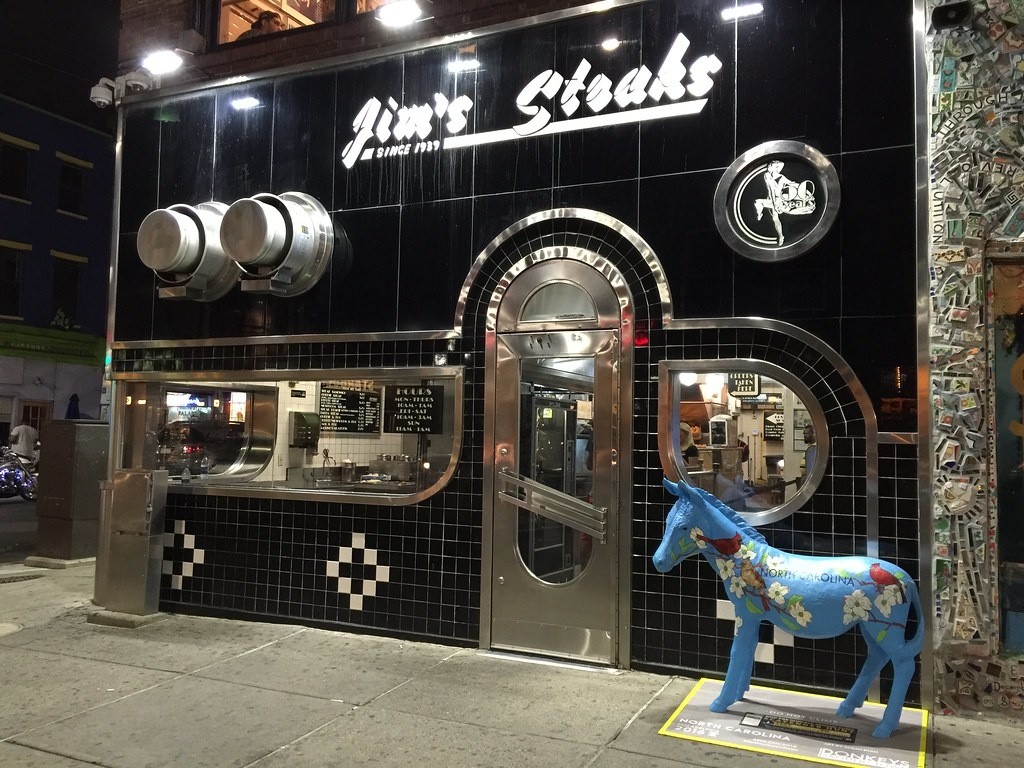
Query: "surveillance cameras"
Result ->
[[124, 72, 152, 94], [89, 84, 112, 109]]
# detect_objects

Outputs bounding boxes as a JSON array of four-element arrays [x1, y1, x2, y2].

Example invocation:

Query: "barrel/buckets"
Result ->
[[342, 462, 356, 481]]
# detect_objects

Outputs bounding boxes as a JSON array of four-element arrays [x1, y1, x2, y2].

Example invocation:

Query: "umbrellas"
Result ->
[[65, 394, 80, 419]]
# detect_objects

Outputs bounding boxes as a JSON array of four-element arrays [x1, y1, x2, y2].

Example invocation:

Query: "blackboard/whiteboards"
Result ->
[[383, 385, 444, 435], [315, 381, 383, 439]]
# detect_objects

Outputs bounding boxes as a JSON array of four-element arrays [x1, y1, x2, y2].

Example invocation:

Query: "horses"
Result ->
[[651, 478, 925, 739]]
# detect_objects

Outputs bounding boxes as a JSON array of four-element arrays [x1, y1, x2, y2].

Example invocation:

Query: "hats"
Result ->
[[680, 422, 691, 432]]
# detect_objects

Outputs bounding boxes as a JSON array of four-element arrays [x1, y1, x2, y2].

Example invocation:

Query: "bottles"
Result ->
[[381, 473, 389, 485], [181, 464, 193, 486], [200, 454, 210, 487]]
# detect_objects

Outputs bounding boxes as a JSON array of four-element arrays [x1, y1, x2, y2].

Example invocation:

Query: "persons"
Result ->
[[235, 11, 283, 44], [580, 433, 594, 568], [800, 425, 816, 480], [9, 416, 39, 461], [680, 421, 699, 464]]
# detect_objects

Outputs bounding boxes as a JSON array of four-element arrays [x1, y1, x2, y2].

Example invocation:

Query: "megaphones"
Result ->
[[931, 0, 973, 31]]
